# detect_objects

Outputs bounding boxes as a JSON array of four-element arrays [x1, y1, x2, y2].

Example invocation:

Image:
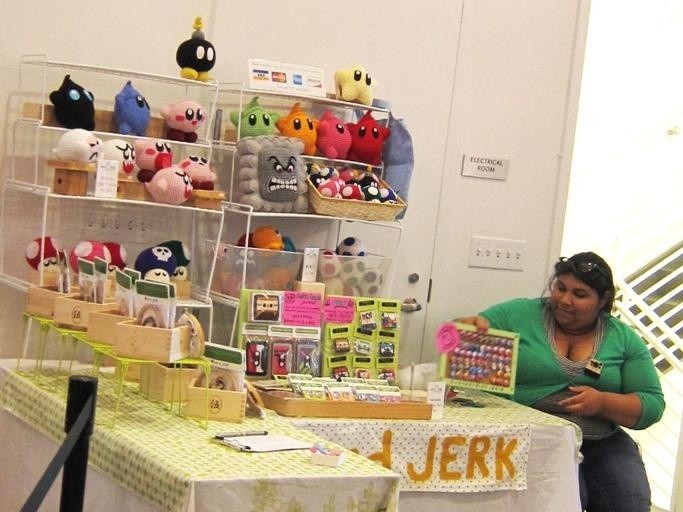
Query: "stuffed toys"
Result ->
[[212, 225, 301, 297], [26, 236, 191, 286], [25, 15, 414, 298], [336, 63, 378, 106], [176, 16, 216, 82], [48, 73, 205, 143], [230, 96, 398, 213], [52, 128, 218, 206]]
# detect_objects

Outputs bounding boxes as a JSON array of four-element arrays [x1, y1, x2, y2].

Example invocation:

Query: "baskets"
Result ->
[[306, 163, 408, 225]]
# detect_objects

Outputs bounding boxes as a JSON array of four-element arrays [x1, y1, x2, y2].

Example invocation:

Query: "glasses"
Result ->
[[555, 256, 610, 280]]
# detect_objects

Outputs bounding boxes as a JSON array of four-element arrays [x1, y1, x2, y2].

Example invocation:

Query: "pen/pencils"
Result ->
[[215, 431, 268, 439]]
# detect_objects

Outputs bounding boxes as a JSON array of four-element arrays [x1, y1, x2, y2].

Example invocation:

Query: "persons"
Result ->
[[451, 252, 666, 512]]
